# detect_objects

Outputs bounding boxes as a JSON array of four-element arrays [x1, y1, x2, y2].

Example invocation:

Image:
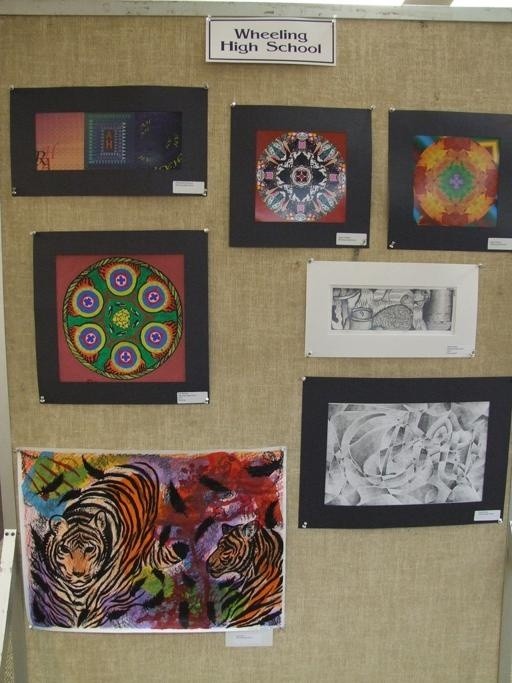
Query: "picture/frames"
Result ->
[[297, 376, 512, 528], [10, 87, 208, 195], [229, 105, 372, 247], [33, 231, 210, 404], [304, 260, 479, 358], [388, 110, 512, 252]]
